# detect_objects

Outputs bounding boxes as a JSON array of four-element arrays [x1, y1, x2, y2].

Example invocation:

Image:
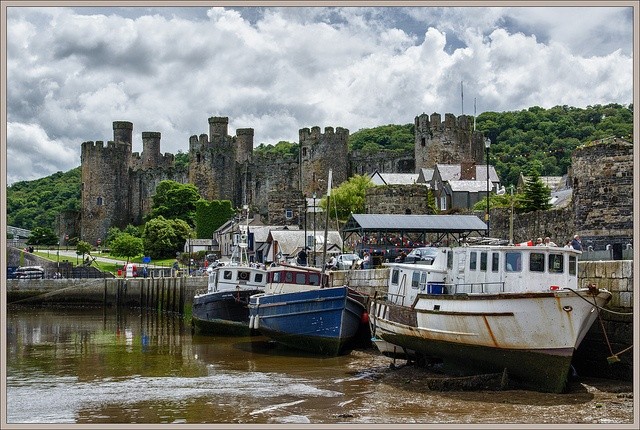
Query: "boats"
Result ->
[[368, 240, 614, 394], [247, 169, 369, 357], [192, 234, 266, 328]]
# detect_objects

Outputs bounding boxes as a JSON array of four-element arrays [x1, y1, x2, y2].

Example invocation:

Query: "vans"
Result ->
[[13, 266, 45, 279]]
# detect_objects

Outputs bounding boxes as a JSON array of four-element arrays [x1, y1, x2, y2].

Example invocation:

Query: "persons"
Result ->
[[571, 234, 581, 250], [398, 250, 407, 263], [329, 256, 338, 268], [360, 252, 370, 270], [536, 238, 545, 247], [544, 237, 557, 247], [296, 249, 307, 266], [564, 240, 575, 250]]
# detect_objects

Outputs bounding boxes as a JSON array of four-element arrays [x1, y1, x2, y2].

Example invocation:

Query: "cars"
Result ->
[[337, 253, 361, 270]]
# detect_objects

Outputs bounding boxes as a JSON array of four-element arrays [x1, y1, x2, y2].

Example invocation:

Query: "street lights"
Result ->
[[484, 138, 492, 235], [313, 191, 318, 267], [189, 233, 191, 275]]
